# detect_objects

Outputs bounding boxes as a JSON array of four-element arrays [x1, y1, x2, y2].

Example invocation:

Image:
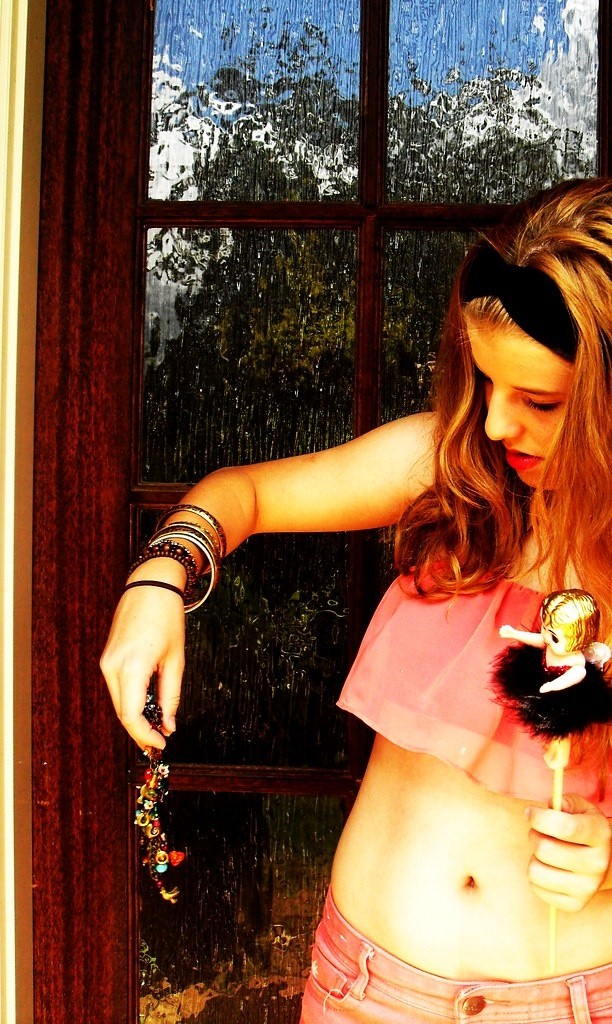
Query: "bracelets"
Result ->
[[127, 520, 222, 614], [154, 503, 227, 560], [122, 580, 185, 602]]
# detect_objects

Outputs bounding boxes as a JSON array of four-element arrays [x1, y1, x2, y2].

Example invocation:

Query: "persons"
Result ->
[[97, 178, 611, 1024], [486, 587, 612, 815]]
